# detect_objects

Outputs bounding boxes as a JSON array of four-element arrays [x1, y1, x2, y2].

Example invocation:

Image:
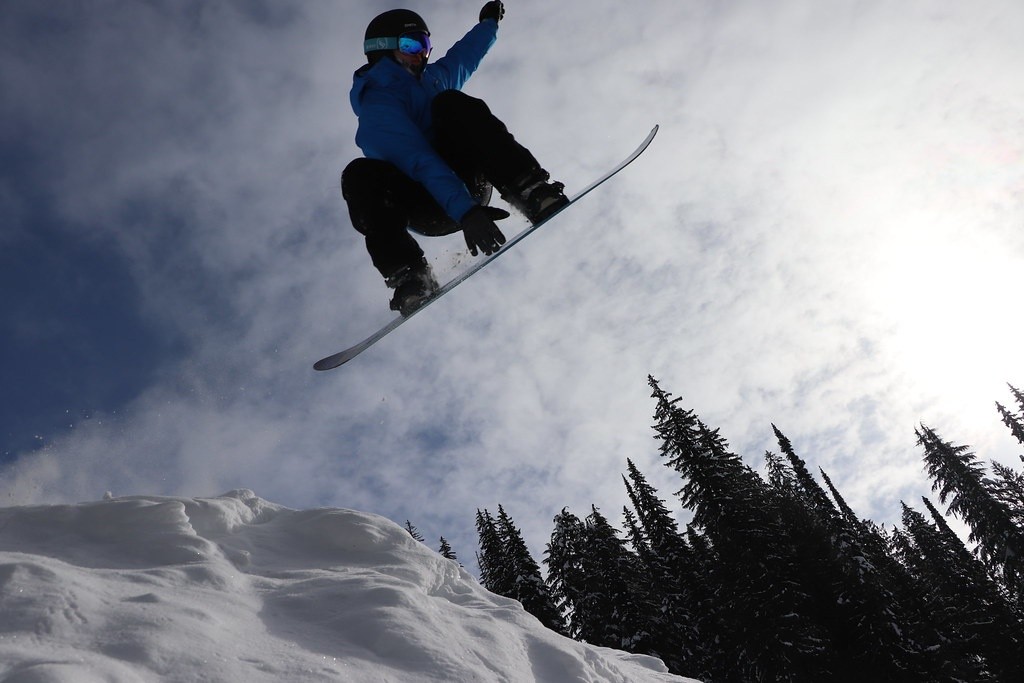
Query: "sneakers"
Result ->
[[521, 182, 570, 225], [390, 267, 444, 318]]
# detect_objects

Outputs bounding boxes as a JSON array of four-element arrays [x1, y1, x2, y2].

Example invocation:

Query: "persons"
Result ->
[[341, 0, 571, 312]]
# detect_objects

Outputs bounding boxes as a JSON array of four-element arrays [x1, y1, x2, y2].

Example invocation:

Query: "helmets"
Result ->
[[364, 9, 431, 78]]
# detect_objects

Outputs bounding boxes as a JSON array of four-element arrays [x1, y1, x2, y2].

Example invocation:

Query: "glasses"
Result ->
[[398, 29, 433, 59]]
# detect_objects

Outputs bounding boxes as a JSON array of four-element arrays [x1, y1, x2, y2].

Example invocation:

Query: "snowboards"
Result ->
[[312, 124, 660, 372]]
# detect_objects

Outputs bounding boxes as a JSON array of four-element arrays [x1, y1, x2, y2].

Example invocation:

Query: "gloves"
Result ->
[[460, 206, 510, 256], [479, 0, 505, 23]]
